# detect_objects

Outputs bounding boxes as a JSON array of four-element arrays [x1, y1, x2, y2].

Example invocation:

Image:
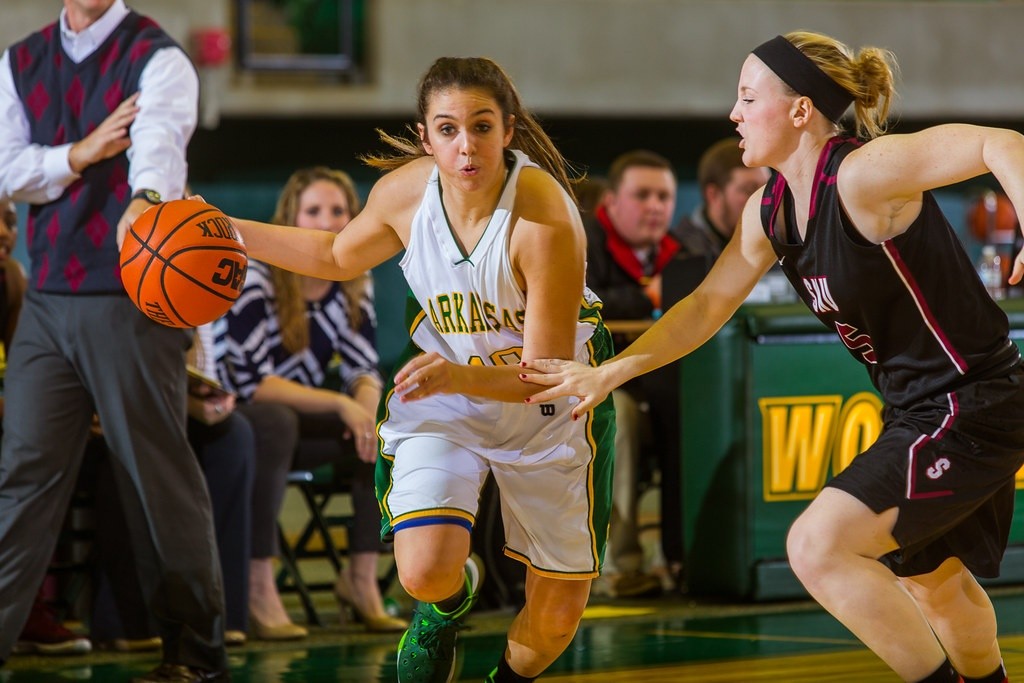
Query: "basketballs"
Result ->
[[119, 200, 249, 328]]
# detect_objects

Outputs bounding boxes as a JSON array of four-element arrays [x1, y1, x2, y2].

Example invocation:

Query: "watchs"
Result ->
[[133, 189, 163, 205]]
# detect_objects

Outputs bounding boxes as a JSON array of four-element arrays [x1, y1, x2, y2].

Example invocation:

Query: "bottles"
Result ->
[[977, 246, 1002, 301]]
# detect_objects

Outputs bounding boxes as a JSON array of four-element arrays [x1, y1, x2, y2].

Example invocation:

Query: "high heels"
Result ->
[[244, 606, 308, 641], [334, 574, 410, 632]]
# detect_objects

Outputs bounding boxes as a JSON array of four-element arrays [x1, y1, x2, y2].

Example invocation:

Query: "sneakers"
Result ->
[[395, 549, 478, 683]]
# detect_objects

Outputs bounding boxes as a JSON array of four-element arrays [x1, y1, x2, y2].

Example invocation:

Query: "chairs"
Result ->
[[275, 434, 362, 627]]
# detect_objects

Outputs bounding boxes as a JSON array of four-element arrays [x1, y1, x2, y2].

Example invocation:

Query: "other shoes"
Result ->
[[13, 620, 91, 652], [97, 636, 163, 648], [593, 571, 662, 599]]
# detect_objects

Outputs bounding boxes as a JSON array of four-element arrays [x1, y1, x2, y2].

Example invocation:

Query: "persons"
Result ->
[[0, 141, 772, 654], [177, 57, 617, 683], [0, 1, 227, 683], [517, 29, 1024, 682]]
